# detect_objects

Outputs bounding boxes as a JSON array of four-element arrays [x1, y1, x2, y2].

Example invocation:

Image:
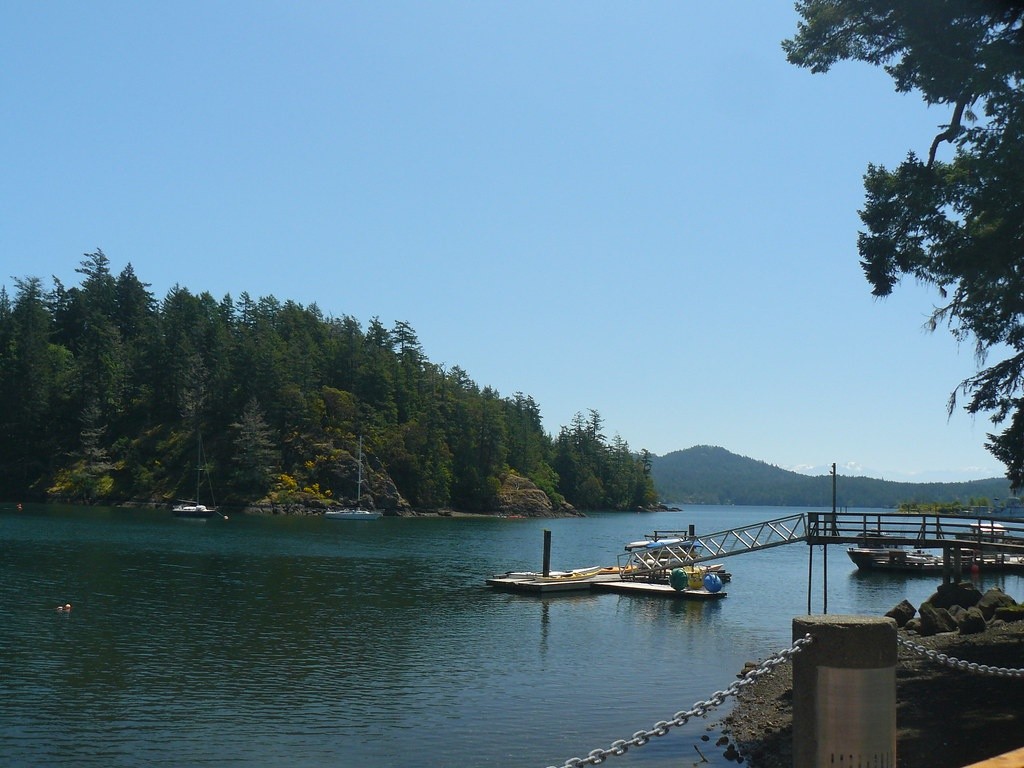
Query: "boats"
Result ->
[[506, 565, 600, 579], [536, 569, 601, 581], [596, 566, 638, 574], [654, 563, 724, 575], [845, 527, 935, 572]]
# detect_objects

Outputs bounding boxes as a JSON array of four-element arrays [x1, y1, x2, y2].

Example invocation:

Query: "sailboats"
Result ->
[[324, 435, 384, 520], [172, 432, 216, 517]]
